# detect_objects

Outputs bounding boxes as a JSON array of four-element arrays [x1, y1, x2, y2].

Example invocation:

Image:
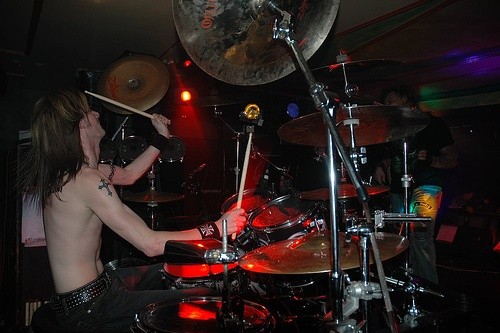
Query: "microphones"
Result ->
[[231, 230, 252, 248]]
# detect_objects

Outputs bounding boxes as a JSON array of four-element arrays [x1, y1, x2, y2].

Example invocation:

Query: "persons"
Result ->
[[374, 85, 455, 283], [14, 86, 247, 333]]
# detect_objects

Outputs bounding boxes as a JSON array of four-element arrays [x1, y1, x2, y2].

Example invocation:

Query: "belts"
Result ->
[[49, 267, 112, 315]]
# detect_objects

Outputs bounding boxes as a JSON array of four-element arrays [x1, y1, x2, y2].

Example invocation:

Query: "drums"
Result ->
[[247, 190, 330, 248], [128, 295, 277, 333], [220, 187, 275, 251], [160, 259, 251, 299]]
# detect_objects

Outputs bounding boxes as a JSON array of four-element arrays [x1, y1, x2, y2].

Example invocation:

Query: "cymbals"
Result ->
[[237, 230, 410, 274], [171, 0, 341, 87], [277, 105, 431, 146], [96, 55, 169, 115], [120, 190, 184, 203], [299, 183, 390, 200], [290, 58, 402, 83]]
